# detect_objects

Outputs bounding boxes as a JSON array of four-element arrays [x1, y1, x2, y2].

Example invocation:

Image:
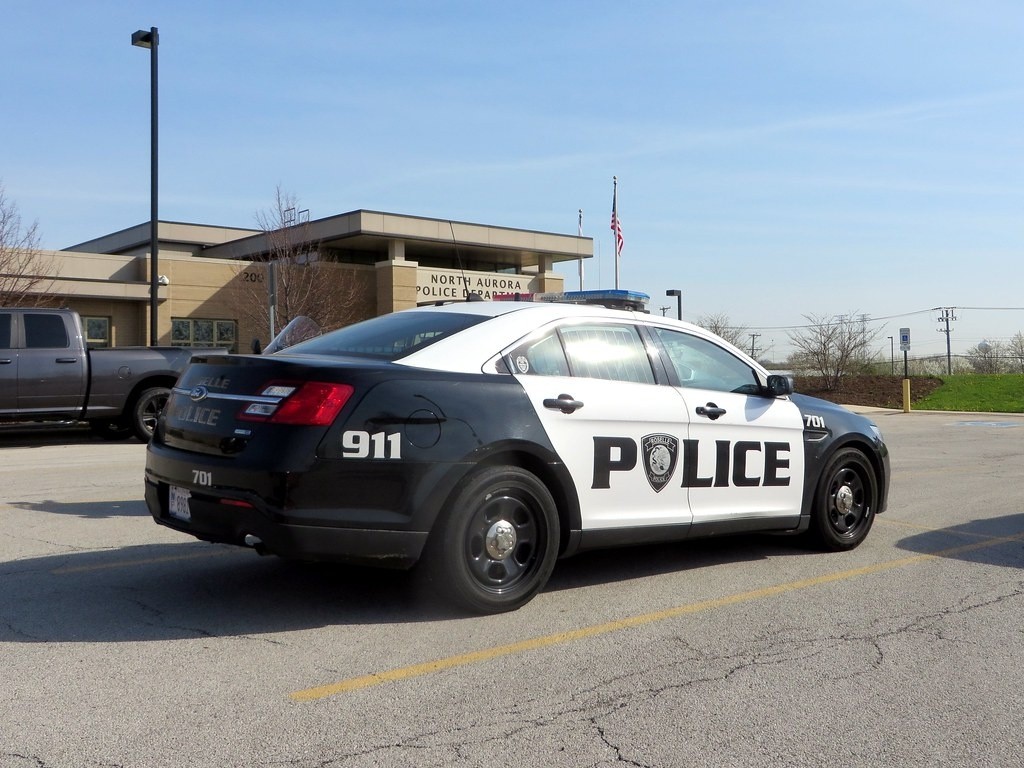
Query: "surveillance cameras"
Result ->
[[162, 278, 169, 286]]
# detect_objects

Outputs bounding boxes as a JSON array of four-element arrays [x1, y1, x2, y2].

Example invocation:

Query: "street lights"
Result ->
[[887, 337, 893, 375], [666, 290, 681, 320], [129, 30, 157, 347]]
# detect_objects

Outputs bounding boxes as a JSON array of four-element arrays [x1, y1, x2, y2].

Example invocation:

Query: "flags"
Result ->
[[578, 226, 585, 277], [611, 191, 624, 256]]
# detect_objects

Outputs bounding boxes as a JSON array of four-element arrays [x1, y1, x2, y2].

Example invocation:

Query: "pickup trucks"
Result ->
[[0, 308, 229, 442]]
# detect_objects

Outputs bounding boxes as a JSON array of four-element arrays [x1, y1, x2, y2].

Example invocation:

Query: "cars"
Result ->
[[145, 293, 893, 615]]
